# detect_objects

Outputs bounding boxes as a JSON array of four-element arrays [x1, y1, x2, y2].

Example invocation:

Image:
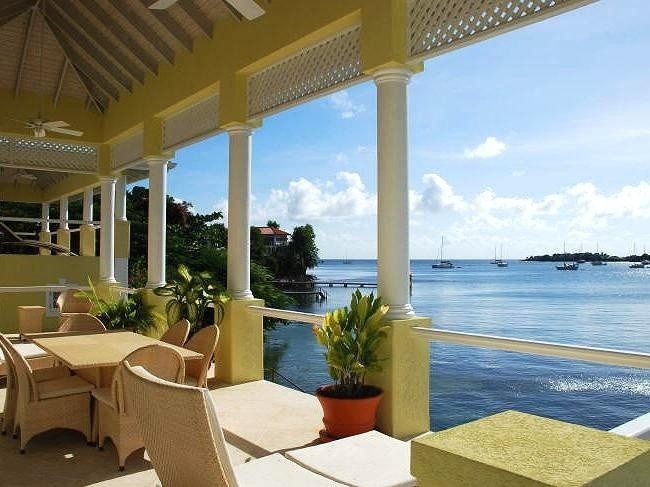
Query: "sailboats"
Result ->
[[591, 241, 607, 265], [498, 244, 508, 266], [432, 234, 455, 268], [490, 246, 503, 264], [555, 239, 579, 270], [628, 242, 650, 269]]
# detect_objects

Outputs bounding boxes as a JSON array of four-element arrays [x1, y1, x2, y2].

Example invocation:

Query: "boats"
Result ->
[[577, 258, 586, 263]]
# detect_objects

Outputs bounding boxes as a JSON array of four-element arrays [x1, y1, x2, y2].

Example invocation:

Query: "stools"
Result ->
[[16, 304, 46, 336]]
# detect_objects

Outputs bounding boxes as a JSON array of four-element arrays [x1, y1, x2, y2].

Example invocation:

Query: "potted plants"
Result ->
[[73, 273, 159, 335], [151, 262, 229, 370], [312, 288, 389, 443]]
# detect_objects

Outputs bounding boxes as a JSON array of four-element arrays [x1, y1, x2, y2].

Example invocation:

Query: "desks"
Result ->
[[30, 328, 206, 445]]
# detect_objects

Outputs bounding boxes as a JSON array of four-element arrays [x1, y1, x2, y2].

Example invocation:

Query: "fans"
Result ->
[[5, 0, 85, 139], [2, 168, 38, 182], [147, 1, 264, 22]]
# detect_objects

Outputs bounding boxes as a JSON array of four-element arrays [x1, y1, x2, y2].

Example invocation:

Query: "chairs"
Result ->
[[0, 333, 96, 455], [0, 337, 73, 434], [159, 318, 192, 347], [56, 288, 96, 317], [122, 361, 239, 487], [57, 311, 108, 336], [180, 324, 222, 386], [88, 344, 187, 473]]
